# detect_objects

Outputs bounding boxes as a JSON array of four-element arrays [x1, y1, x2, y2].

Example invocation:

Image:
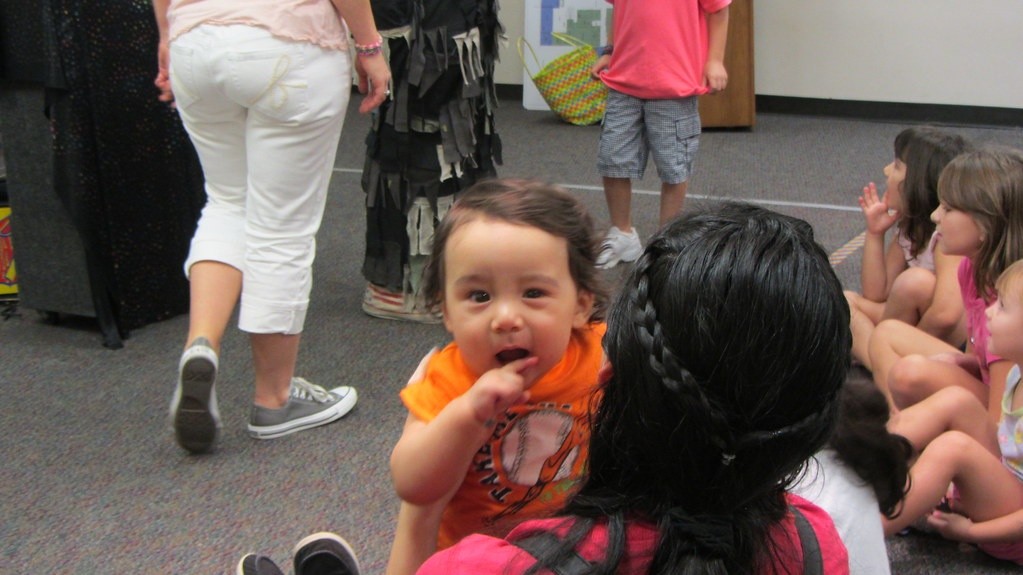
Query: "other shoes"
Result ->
[[292, 533, 359, 574], [236, 551, 284, 574]]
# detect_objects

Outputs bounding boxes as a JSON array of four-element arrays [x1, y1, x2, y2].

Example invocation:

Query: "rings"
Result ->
[[384, 90, 391, 96]]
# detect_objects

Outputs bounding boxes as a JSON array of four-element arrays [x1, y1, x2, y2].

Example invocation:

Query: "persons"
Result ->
[[841, 124, 978, 375], [389, 180, 610, 546], [358, 0, 504, 324], [868, 145, 1023, 414], [873, 258, 1023, 565], [144, 0, 393, 454], [235, 204, 853, 575], [590, 0, 735, 270], [786, 359, 917, 575]]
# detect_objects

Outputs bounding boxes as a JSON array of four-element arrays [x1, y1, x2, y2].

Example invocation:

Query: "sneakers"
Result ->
[[363, 282, 446, 327], [246, 378, 358, 440], [595, 226, 644, 270], [170, 336, 220, 453]]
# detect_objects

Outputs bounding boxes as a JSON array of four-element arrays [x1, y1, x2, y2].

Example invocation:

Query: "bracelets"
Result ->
[[600, 45, 613, 55], [355, 33, 384, 57]]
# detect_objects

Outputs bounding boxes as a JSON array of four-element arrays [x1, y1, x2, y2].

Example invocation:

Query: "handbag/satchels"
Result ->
[[516, 31, 608, 125]]
